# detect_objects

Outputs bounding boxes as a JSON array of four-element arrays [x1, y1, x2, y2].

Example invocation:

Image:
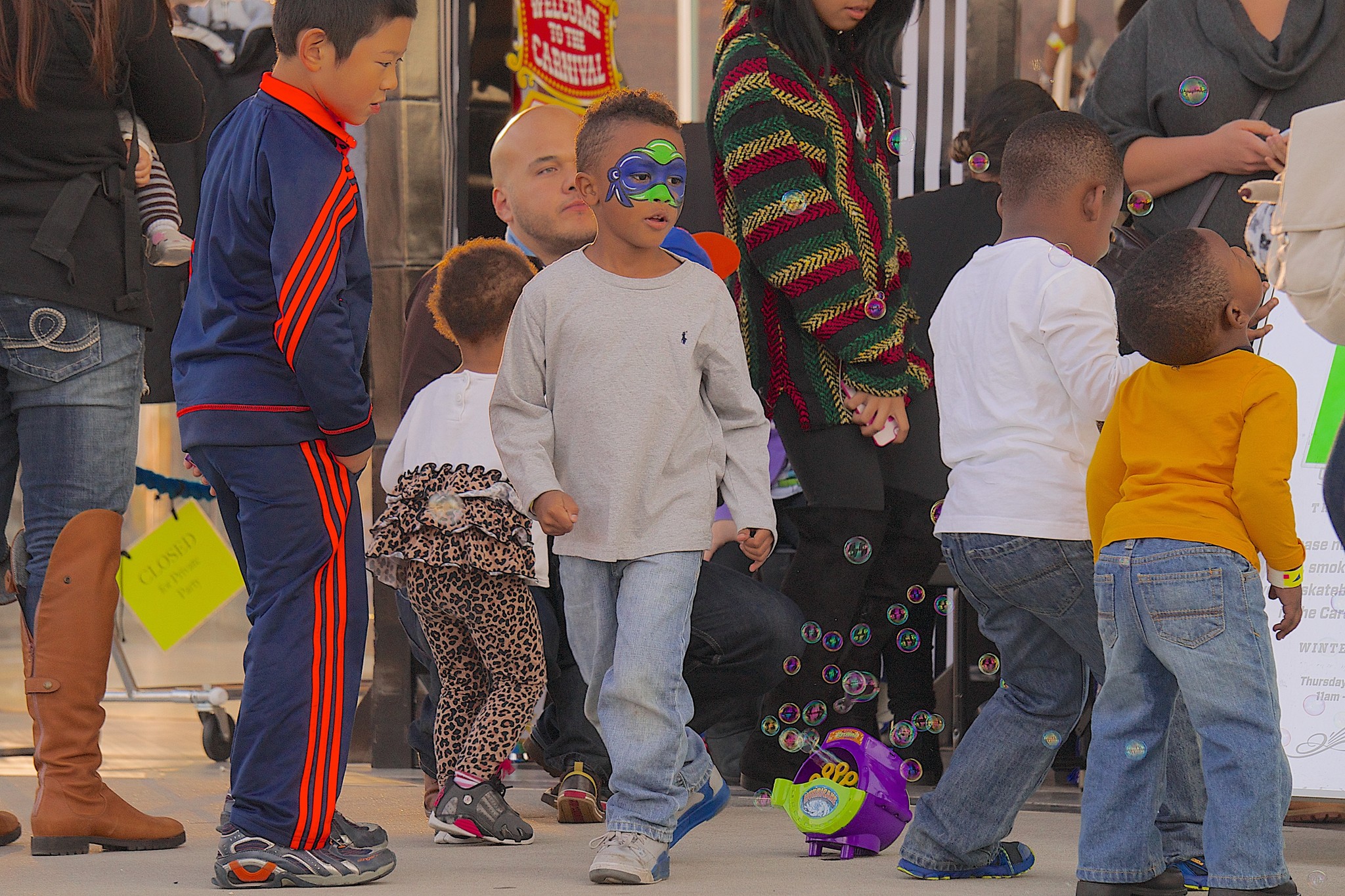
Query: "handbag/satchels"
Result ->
[[1093, 226, 1152, 356]]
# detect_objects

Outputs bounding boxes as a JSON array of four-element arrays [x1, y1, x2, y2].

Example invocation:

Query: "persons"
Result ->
[[363, 238, 552, 849], [0, 0, 806, 887], [712, 0, 934, 794], [875, 0, 1345, 896]]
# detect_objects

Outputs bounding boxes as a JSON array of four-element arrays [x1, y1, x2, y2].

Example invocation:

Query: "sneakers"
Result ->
[[589, 831, 670, 884], [557, 761, 605, 823], [424, 772, 440, 817], [666, 767, 730, 849], [1166, 856, 1209, 890], [433, 829, 487, 844], [211, 821, 397, 890], [541, 783, 608, 811], [428, 774, 534, 845], [896, 841, 1036, 880], [215, 789, 389, 852]]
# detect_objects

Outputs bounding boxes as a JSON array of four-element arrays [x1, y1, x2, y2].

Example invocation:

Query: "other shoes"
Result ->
[[907, 769, 942, 786], [522, 733, 565, 778], [147, 229, 195, 267], [1283, 800, 1345, 822], [1078, 770, 1086, 792], [1076, 866, 1187, 896], [1054, 769, 1077, 787], [1208, 875, 1301, 896]]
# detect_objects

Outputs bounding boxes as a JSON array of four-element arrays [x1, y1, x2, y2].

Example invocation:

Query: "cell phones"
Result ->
[[840, 379, 899, 447]]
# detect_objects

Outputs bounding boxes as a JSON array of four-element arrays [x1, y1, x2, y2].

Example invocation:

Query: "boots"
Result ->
[[0, 811, 21, 846], [740, 502, 890, 799], [833, 485, 945, 745], [11, 509, 187, 856]]
